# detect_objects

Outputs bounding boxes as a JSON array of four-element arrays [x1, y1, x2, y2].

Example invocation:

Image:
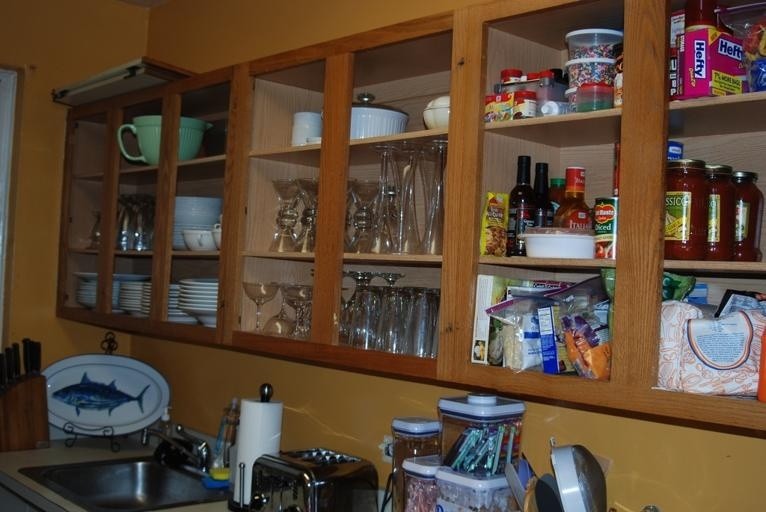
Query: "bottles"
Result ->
[[555, 167, 594, 232], [508, 156, 539, 255], [551, 177, 566, 209], [531, 162, 554, 229]]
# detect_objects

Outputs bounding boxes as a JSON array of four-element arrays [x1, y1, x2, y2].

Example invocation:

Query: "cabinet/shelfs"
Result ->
[[54, 63, 237, 347], [220, 7, 466, 383], [454, 2, 766, 432]]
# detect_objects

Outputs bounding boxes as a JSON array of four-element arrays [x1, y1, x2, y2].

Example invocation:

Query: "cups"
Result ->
[[348, 285, 441, 359], [117, 192, 154, 250], [90, 210, 101, 240], [211, 223, 221, 250], [182, 230, 218, 251]]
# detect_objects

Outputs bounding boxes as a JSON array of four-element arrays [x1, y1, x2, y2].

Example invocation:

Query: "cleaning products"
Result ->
[[209, 397, 240, 468]]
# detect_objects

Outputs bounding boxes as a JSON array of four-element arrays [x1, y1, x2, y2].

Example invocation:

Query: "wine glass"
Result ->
[[262, 284, 295, 338], [280, 284, 312, 340], [366, 139, 448, 254], [339, 272, 405, 340], [243, 280, 279, 333]]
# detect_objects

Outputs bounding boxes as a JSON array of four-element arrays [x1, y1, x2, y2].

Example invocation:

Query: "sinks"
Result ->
[[11, 455, 238, 510]]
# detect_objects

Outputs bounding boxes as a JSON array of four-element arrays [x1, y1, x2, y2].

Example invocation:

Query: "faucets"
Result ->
[[142, 423, 210, 471]]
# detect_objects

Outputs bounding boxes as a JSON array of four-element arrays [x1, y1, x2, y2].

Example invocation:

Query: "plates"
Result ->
[[119, 280, 143, 314], [170, 196, 223, 230], [113, 273, 150, 281], [111, 279, 124, 313], [176, 277, 219, 328], [72, 270, 98, 307], [142, 281, 153, 314], [39, 353, 171, 438], [167, 283, 197, 324]]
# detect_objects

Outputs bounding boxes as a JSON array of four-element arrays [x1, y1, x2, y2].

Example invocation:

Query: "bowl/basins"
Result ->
[[349, 91, 411, 139], [117, 114, 213, 165]]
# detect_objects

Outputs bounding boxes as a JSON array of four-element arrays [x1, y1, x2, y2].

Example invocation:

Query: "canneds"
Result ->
[[664, 159, 707, 258], [703, 164, 733, 260], [733, 169, 764, 262], [593, 196, 619, 257]]
